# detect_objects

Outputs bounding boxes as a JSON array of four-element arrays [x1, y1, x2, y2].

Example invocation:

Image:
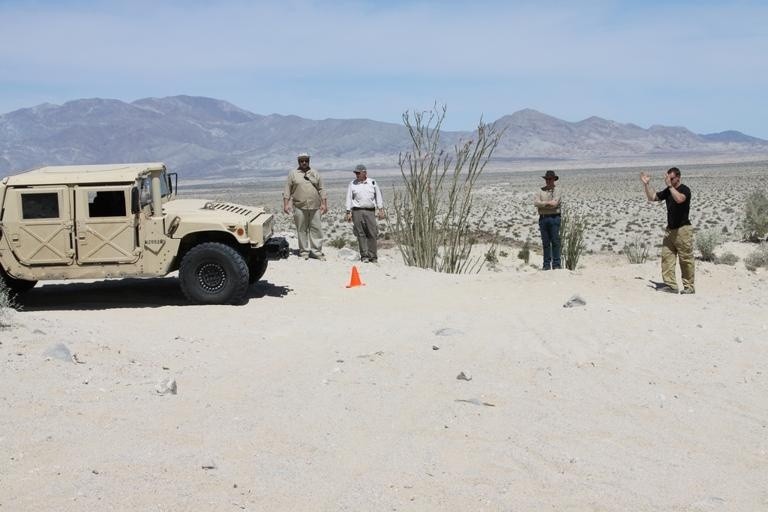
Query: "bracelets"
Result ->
[[346, 210, 352, 215], [668, 184, 673, 189]]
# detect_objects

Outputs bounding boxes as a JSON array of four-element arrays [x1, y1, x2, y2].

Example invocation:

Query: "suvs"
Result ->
[[0, 162, 290, 305]]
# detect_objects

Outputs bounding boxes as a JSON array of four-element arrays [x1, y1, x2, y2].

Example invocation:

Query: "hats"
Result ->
[[296, 152, 311, 160], [352, 164, 368, 174], [540, 170, 558, 181]]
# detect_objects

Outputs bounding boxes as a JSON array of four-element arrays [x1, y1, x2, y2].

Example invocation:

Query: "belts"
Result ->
[[352, 207, 375, 211]]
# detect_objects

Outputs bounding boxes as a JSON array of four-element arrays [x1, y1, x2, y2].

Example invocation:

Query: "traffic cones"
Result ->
[[346, 266, 365, 288]]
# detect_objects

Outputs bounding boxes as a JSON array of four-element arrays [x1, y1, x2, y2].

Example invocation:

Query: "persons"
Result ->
[[281, 152, 328, 261], [531, 168, 564, 271], [344, 164, 385, 264], [639, 166, 696, 294]]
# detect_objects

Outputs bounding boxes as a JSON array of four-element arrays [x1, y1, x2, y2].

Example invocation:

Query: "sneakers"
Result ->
[[299, 251, 327, 263], [680, 287, 695, 295], [655, 283, 679, 295], [360, 256, 378, 265]]
